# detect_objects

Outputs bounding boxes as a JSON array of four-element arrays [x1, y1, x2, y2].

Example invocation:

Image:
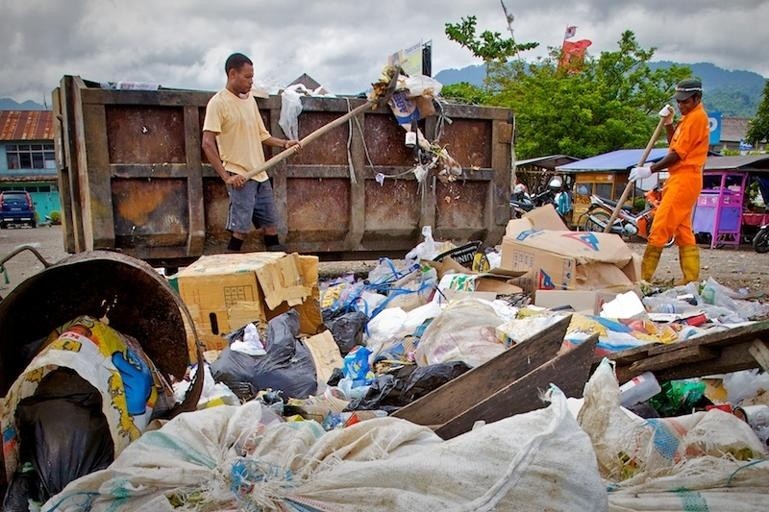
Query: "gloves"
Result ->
[[629, 166, 652, 183], [658, 104, 675, 126]]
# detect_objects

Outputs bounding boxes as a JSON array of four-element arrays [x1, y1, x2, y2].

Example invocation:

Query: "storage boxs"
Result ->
[[176, 252, 322, 366], [494, 199, 643, 293]]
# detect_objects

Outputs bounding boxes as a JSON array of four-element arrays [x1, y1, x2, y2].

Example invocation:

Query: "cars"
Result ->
[[0, 190, 38, 229]]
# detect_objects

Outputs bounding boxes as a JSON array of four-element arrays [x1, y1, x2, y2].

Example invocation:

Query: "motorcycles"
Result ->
[[510, 179, 676, 249]]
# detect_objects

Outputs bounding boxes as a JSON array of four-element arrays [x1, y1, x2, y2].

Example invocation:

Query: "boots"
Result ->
[[638, 244, 663, 283], [670, 245, 701, 287]]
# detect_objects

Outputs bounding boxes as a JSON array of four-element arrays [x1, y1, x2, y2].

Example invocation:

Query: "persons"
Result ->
[[577, 183, 588, 196], [554, 183, 572, 230], [566, 185, 574, 204], [628, 79, 711, 287], [201, 52, 306, 253], [514, 176, 528, 195]]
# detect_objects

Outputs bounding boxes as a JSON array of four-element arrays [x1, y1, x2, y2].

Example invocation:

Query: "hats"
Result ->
[[668, 78, 704, 102]]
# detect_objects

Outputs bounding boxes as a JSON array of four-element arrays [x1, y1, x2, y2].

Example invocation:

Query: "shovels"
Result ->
[[233, 66, 414, 188]]
